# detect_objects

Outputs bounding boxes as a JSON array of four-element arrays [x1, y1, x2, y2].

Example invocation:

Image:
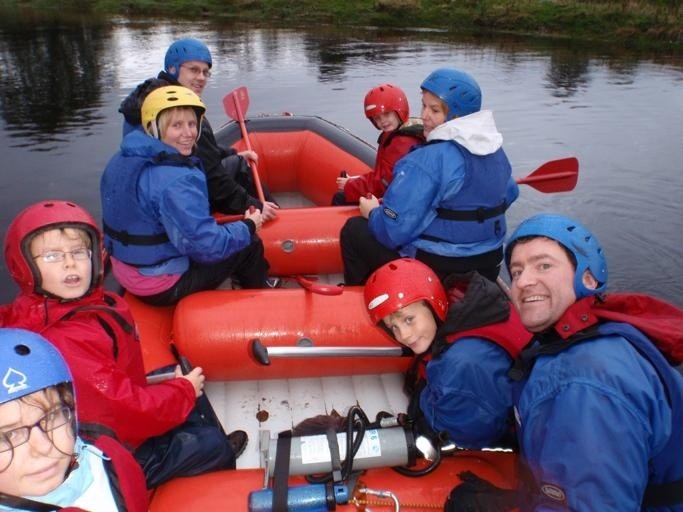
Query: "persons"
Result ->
[[0, 199, 250, 490], [330, 84, 428, 206], [118, 37, 279, 223], [98, 85, 281, 307], [504, 214, 682, 511], [0, 327, 151, 511], [365, 259, 535, 448], [339, 69, 520, 285]]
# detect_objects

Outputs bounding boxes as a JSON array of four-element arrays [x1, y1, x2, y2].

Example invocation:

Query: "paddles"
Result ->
[[366, 158, 579, 206], [221, 86, 266, 205]]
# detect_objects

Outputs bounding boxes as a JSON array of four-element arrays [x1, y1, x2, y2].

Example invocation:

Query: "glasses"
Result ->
[[32, 248, 91, 262], [180, 65, 210, 78], [0, 405, 71, 453]]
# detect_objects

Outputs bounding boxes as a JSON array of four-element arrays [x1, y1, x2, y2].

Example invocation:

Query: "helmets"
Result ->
[[420, 69, 481, 123], [164, 39, 211, 80], [364, 84, 409, 130], [504, 213, 608, 298], [364, 257, 449, 349], [140, 85, 205, 143], [0, 327, 77, 434], [3, 200, 104, 296]]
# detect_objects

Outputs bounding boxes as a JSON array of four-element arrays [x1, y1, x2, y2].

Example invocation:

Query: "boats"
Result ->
[[101, 114, 538, 510]]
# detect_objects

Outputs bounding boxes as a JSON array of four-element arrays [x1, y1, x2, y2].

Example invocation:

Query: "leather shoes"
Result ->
[[226, 430, 248, 458]]
